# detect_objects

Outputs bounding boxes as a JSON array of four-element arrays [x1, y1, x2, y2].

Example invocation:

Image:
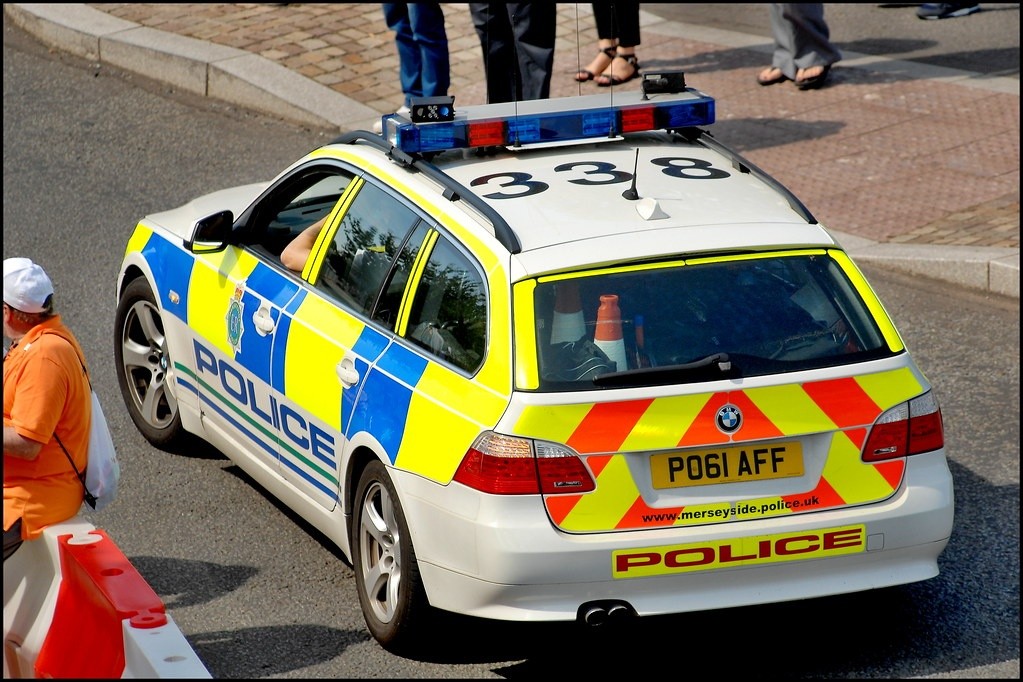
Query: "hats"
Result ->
[[2, 255, 53, 313]]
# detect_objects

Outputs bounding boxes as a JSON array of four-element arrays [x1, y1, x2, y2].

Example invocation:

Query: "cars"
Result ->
[[115, 83, 961, 650]]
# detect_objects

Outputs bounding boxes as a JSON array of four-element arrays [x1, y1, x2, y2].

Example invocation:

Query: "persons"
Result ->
[[469, 1, 557, 106], [374, 2, 453, 135], [574, 3, 642, 89], [3, 257, 96, 565], [280, 211, 332, 274], [916, 3, 981, 21], [753, 2, 843, 88]]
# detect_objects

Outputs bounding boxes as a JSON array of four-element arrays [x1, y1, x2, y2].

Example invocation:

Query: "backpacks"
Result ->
[[20, 332, 119, 513]]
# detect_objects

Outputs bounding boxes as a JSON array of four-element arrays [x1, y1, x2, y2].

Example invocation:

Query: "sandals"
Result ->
[[574, 46, 619, 81], [594, 53, 640, 86]]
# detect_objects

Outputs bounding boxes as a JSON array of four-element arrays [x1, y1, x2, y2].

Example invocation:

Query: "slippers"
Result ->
[[793, 58, 832, 87], [755, 66, 786, 86]]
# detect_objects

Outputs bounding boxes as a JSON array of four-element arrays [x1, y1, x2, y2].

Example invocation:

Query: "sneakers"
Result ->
[[373, 106, 411, 132], [915, 3, 981, 19]]
